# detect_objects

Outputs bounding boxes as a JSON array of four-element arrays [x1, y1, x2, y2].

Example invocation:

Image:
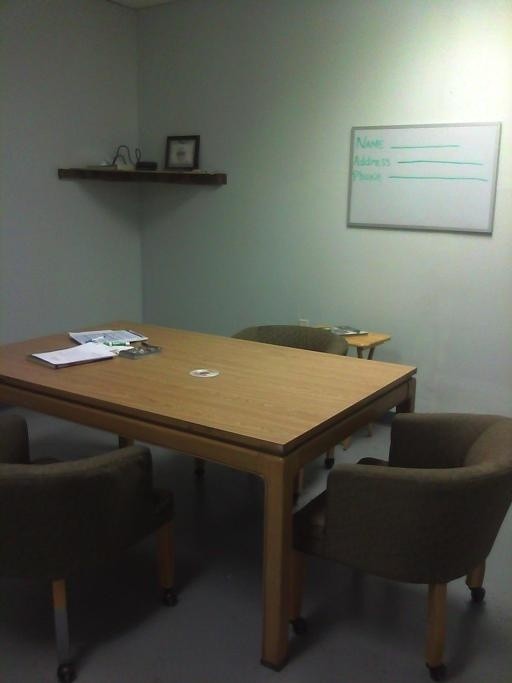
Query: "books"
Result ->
[[67, 327, 151, 344], [319, 324, 368, 336], [29, 340, 119, 371]]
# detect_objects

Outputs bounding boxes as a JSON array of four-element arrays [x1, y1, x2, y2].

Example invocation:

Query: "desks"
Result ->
[[2, 322, 419, 674], [344, 331, 391, 450]]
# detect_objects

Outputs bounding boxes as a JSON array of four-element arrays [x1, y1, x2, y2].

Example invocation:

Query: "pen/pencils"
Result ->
[[85, 336, 130, 346]]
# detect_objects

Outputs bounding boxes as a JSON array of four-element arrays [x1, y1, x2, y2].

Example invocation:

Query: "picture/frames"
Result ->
[[163, 134, 201, 172]]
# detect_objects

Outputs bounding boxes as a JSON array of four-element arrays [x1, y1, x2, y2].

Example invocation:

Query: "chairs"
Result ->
[[289, 408, 510, 682], [0, 408, 177, 682], [195, 322, 347, 503]]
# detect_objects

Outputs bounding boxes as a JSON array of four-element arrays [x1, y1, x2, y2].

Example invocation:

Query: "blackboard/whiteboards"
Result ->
[[346, 121, 502, 235]]
